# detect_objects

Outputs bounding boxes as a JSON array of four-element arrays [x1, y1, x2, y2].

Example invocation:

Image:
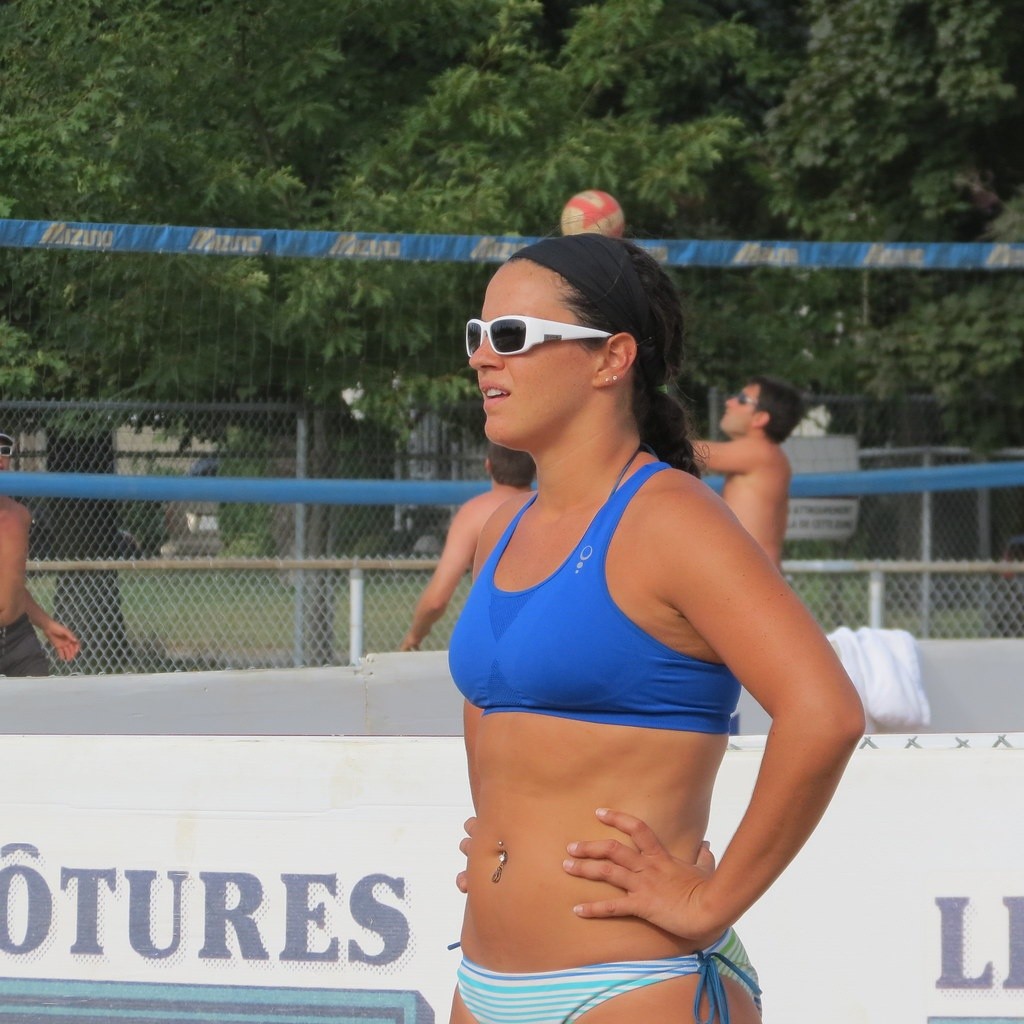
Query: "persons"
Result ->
[[449, 234, 865, 1024], [0, 429, 80, 677], [400, 443, 536, 650], [691, 375, 800, 582]]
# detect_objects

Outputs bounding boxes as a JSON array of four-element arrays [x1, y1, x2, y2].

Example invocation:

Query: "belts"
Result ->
[[0, 613, 28, 637]]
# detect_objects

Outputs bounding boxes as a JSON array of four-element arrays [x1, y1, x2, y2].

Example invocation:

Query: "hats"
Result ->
[[0, 433, 13, 446]]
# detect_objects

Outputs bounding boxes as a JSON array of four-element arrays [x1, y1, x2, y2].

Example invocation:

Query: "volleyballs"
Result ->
[[561, 188, 626, 239]]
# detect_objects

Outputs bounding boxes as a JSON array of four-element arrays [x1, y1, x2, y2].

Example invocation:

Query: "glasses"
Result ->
[[0, 444, 15, 457], [737, 393, 760, 406], [466, 316, 613, 358]]
